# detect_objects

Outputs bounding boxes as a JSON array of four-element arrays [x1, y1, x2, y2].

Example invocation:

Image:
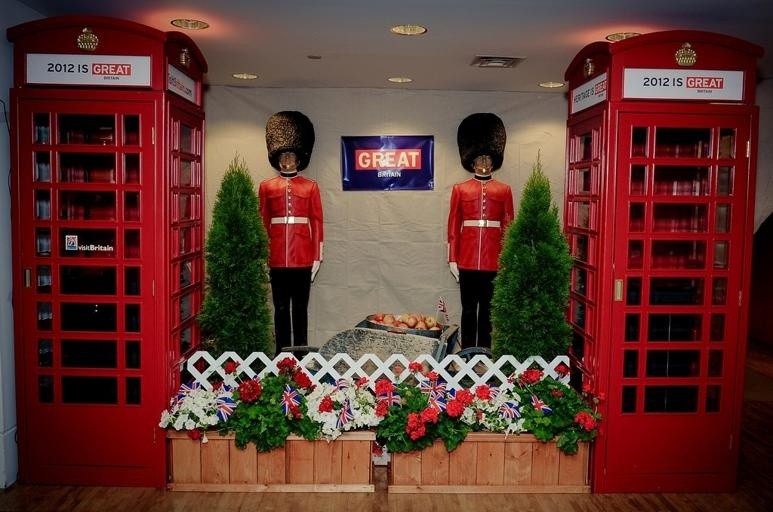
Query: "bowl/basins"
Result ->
[[364, 313, 443, 338]]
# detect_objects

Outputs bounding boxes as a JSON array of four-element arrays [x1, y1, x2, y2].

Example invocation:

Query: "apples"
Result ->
[[371, 313, 441, 331]]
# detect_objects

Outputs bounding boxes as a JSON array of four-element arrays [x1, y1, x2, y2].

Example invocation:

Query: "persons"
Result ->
[[258, 111, 324, 362], [446, 113, 514, 363]]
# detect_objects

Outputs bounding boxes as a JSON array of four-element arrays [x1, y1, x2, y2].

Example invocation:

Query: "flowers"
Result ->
[[160, 357, 603, 455]]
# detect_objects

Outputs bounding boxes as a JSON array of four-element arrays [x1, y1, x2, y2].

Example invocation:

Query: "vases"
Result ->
[[159, 420, 377, 497], [381, 420, 594, 497]]
